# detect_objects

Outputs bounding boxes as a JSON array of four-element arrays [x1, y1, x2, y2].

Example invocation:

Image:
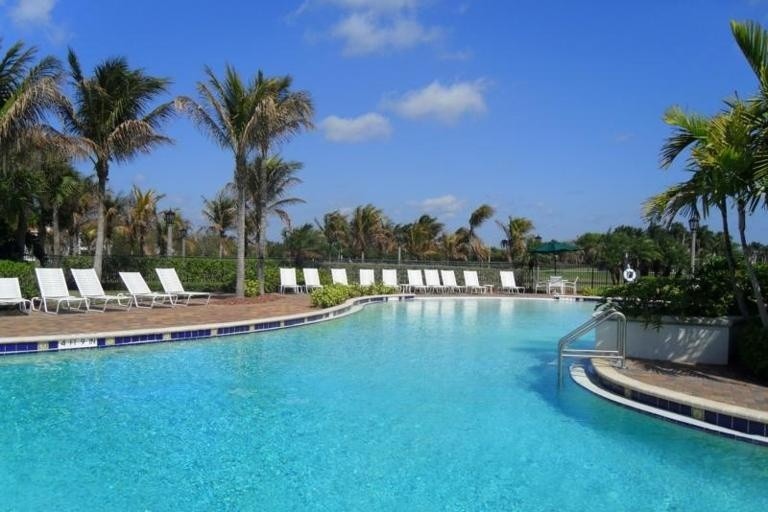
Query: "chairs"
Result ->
[[531, 272, 581, 296], [1, 265, 213, 316], [274, 266, 530, 297]]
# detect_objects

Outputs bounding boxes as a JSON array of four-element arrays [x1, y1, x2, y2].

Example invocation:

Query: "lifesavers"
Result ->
[[623, 268, 636, 282]]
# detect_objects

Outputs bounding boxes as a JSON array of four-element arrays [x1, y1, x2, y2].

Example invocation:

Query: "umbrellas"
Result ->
[[528, 240, 583, 276]]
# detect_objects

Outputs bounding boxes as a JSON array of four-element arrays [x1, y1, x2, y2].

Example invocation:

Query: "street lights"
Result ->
[[164, 206, 176, 256], [688, 218, 699, 274], [180, 228, 187, 256]]
